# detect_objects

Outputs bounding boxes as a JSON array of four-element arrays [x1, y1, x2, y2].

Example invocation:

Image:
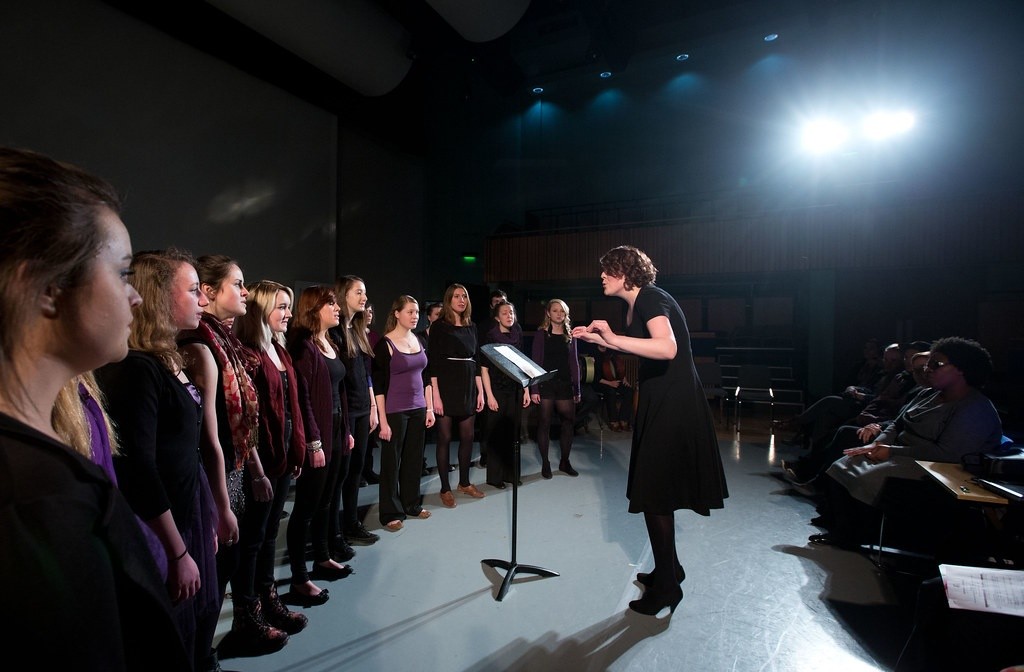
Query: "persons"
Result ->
[[571, 247, 729, 615], [370, 295, 435, 532], [230, 280, 309, 655], [416, 284, 486, 507], [588, 344, 634, 432], [176, 256, 260, 672], [479, 289, 531, 490], [770, 336, 1003, 550], [328, 275, 380, 563], [93, 251, 219, 672], [531, 299, 581, 479], [288, 285, 354, 606], [0, 147, 193, 672]]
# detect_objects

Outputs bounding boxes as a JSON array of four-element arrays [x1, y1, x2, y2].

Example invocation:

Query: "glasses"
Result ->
[[923, 361, 954, 367]]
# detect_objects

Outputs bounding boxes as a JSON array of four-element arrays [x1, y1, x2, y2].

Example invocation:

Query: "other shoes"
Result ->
[[780, 458, 859, 549], [620, 419, 632, 431], [610, 421, 623, 432], [772, 416, 803, 432], [230, 458, 582, 646], [780, 434, 810, 450]]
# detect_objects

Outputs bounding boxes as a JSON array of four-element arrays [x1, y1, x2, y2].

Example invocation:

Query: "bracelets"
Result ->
[[427, 410, 432, 411], [371, 404, 376, 407], [307, 440, 322, 454], [168, 546, 188, 561], [253, 475, 266, 482]]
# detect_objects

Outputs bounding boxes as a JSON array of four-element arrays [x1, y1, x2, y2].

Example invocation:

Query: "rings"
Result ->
[[228, 539, 233, 542]]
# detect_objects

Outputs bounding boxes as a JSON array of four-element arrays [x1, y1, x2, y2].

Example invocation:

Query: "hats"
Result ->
[[898, 340, 930, 351]]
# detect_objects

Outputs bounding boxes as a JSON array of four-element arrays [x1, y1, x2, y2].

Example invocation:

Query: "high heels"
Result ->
[[628, 583, 682, 616], [637, 566, 685, 585]]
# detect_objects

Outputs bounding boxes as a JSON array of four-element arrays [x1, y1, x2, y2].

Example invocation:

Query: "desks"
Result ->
[[893, 459, 1019, 672]]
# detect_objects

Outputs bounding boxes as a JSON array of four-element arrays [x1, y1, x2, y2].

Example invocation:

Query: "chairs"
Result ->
[[592, 390, 620, 430], [870, 432, 1014, 569], [696, 362, 727, 424]]
